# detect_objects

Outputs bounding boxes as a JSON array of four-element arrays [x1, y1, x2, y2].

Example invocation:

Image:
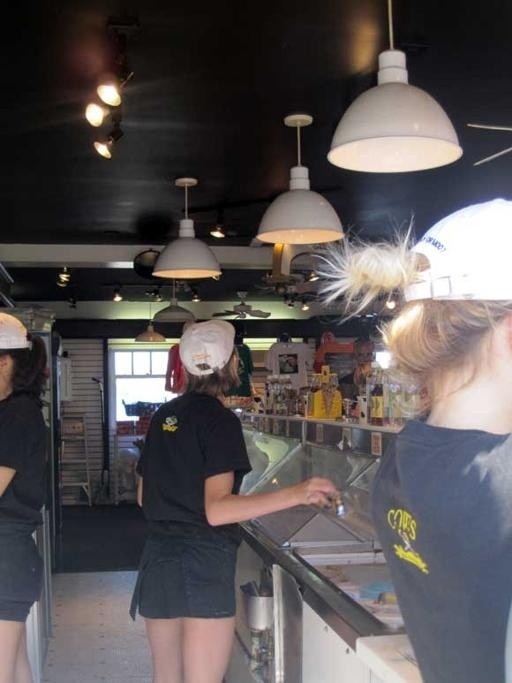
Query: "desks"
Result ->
[[349, 630, 424, 683]]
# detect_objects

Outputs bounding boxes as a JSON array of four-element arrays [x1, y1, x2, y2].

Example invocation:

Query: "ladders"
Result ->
[[59, 415, 92, 507]]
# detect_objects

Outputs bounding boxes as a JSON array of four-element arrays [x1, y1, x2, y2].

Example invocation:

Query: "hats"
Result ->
[[1, 313, 31, 351], [402, 199, 511, 304], [179, 320, 237, 376]]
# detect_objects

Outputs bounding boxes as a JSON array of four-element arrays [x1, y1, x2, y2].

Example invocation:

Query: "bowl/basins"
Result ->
[[243, 592, 274, 631]]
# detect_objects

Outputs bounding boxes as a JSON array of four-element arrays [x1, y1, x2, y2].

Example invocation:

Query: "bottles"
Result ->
[[263, 373, 294, 415], [368, 369, 383, 424]]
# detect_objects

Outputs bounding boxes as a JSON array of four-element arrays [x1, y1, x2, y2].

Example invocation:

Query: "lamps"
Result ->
[[112, 280, 199, 304], [319, 1, 467, 178], [76, 11, 149, 161], [280, 268, 326, 313], [135, 304, 166, 343], [150, 279, 196, 323], [152, 177, 223, 279], [256, 115, 347, 245]]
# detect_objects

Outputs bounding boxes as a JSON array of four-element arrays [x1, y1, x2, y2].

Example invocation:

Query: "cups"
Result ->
[[357, 395, 368, 424], [334, 398, 359, 425]]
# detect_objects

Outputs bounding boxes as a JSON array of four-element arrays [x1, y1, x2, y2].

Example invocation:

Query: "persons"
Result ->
[[0, 308, 48, 683], [129, 319, 339, 683], [336, 336, 383, 416], [312, 197, 512, 683]]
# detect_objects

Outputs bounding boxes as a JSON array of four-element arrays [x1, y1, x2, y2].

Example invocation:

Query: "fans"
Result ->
[[210, 289, 273, 321]]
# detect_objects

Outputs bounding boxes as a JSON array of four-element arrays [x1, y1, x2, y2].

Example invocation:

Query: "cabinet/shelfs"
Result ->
[[231, 413, 422, 683]]
